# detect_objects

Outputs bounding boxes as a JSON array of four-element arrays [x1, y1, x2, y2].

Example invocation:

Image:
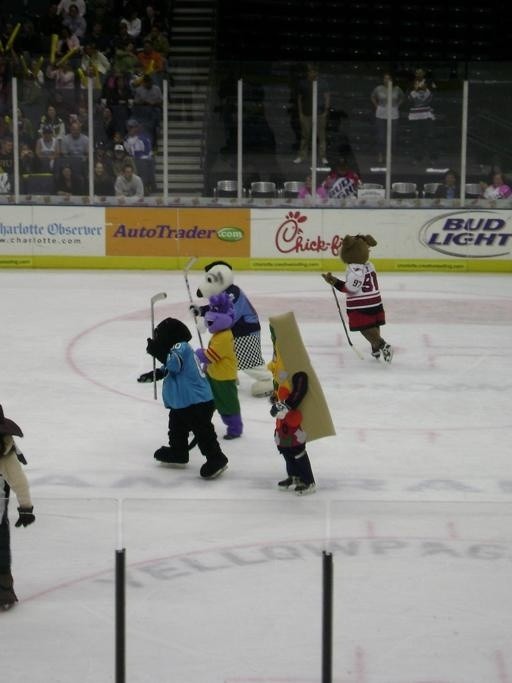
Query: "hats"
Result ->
[[42, 126, 53, 134], [113, 144, 125, 151]]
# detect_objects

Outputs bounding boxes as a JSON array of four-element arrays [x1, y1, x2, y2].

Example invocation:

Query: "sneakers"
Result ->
[[371, 345, 392, 363], [294, 158, 328, 164], [223, 434, 241, 439], [294, 482, 315, 491], [278, 477, 294, 486]]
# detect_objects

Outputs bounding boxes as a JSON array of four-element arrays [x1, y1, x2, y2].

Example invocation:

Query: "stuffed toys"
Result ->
[[1, 405, 35, 609], [268, 311, 336, 490], [194, 291, 243, 440], [320, 234, 392, 362], [136, 317, 228, 478], [190, 261, 275, 398]]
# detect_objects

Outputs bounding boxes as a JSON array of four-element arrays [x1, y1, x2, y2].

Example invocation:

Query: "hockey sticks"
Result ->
[[332, 283, 364, 361], [152, 293, 167, 401], [183, 256, 203, 348]]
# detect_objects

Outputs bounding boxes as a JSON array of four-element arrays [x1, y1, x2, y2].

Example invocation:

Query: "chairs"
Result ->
[[0, 0, 164, 197]]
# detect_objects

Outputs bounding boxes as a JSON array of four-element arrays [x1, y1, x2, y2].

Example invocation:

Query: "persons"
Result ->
[[434, 169, 460, 199], [321, 158, 362, 199], [292, 63, 332, 165], [479, 166, 512, 190], [370, 73, 407, 164], [406, 67, 438, 166], [0, 1, 174, 200], [482, 171, 512, 199], [298, 174, 329, 203]]
